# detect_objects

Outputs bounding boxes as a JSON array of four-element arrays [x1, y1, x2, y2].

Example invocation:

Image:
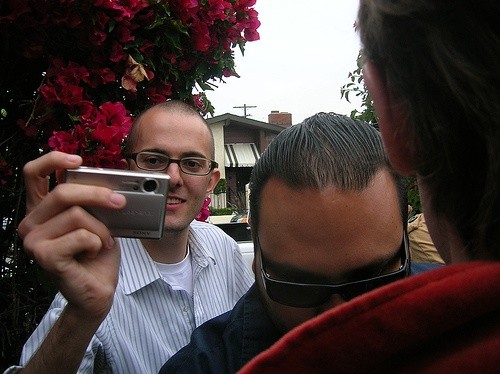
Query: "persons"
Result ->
[[157, 112, 448, 374], [5, 101, 255, 374], [215, 0, 500, 374]]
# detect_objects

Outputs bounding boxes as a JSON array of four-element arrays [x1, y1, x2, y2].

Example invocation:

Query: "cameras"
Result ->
[[62, 165, 171, 239]]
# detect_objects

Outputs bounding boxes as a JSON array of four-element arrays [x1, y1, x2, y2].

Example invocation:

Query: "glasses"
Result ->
[[125, 151, 219, 176], [254, 228, 412, 308]]
